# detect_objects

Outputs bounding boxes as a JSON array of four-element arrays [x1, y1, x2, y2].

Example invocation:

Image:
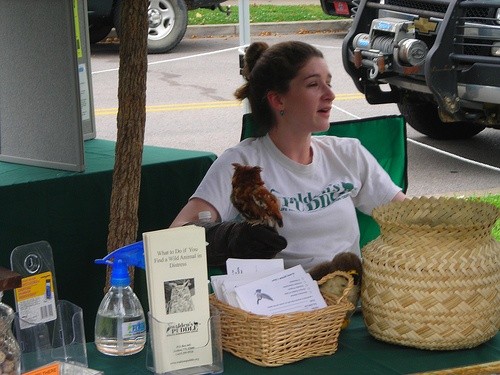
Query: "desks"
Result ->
[[0, 139, 217, 352], [21, 306, 500, 375]]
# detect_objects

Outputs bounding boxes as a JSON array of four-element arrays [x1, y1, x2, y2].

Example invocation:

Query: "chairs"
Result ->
[[240, 114, 407, 255]]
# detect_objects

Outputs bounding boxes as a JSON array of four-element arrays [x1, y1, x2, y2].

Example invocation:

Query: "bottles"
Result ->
[[0, 290, 22, 375], [199, 211, 213, 229]]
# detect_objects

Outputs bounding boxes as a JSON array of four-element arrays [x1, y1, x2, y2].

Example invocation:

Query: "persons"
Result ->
[[168, 41, 409, 274]]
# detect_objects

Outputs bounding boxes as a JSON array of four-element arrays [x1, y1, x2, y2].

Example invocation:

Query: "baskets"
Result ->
[[209, 271, 355, 366]]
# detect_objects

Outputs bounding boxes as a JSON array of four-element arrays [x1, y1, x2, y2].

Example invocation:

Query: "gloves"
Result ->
[[184, 220, 289, 266]]
